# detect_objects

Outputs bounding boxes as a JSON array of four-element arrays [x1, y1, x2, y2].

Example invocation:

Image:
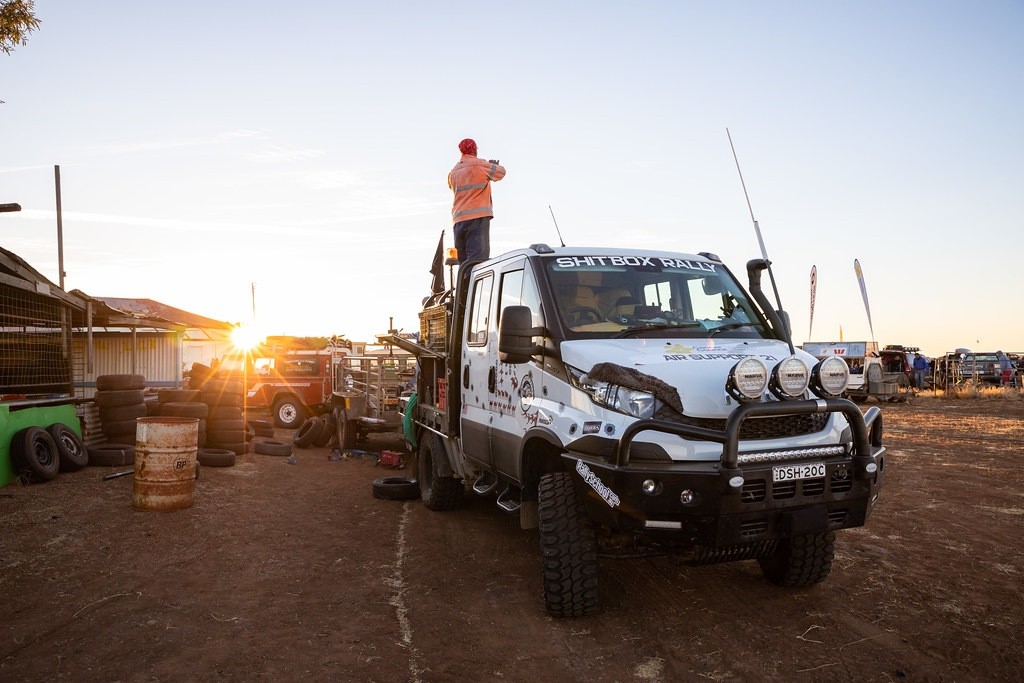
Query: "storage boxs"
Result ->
[[382, 449, 407, 468], [438, 376, 445, 410]]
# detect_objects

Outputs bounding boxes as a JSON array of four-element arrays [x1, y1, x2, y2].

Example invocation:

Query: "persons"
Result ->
[[931, 358, 938, 377], [913, 353, 928, 391], [941, 356, 951, 388], [995, 350, 1013, 386], [448, 139, 506, 266]]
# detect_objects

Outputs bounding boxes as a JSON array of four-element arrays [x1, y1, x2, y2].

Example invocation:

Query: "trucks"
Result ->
[[182, 336, 353, 429], [375, 244, 886, 620]]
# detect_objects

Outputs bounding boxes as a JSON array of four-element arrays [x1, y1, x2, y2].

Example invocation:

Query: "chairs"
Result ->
[[565, 287, 643, 326]]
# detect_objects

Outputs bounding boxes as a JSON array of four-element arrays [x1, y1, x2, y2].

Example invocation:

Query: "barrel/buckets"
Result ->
[[132, 416, 199, 510]]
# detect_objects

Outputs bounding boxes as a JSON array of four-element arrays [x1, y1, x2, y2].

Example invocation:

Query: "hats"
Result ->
[[458, 139, 479, 154]]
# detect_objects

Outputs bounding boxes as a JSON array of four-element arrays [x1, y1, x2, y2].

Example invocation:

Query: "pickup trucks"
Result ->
[[879, 345, 1020, 386]]
